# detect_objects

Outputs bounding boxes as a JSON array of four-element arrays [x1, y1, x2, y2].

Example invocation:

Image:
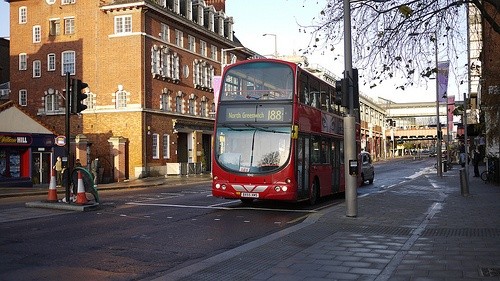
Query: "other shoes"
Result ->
[[473, 175, 477, 177]]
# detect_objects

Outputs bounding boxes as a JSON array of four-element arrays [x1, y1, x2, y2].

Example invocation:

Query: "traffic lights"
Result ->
[[70, 78, 89, 114]]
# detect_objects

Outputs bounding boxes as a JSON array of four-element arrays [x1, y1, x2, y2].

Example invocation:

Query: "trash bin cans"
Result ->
[[459, 168, 468, 194], [444, 160, 448, 172]]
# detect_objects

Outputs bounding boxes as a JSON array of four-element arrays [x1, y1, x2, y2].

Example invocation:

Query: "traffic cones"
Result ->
[[40, 166, 60, 204], [73, 170, 92, 206]]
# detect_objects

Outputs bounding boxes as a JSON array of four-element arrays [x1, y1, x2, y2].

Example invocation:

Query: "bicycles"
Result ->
[[479, 150, 500, 185]]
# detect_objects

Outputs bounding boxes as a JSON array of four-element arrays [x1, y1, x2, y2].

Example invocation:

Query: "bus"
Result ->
[[210, 58, 362, 206]]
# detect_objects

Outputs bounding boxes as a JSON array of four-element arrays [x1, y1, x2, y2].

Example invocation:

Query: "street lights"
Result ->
[[221, 47, 243, 75], [263, 33, 277, 58]]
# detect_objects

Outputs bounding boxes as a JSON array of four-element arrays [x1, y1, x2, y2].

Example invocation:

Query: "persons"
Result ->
[[74, 159, 82, 168], [91, 157, 99, 186], [53, 156, 65, 187], [459, 150, 481, 177]]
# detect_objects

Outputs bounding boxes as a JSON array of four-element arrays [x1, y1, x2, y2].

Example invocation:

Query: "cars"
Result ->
[[361, 150, 375, 185]]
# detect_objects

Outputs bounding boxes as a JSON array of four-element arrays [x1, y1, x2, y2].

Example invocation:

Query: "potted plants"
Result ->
[[200, 149, 208, 172]]
